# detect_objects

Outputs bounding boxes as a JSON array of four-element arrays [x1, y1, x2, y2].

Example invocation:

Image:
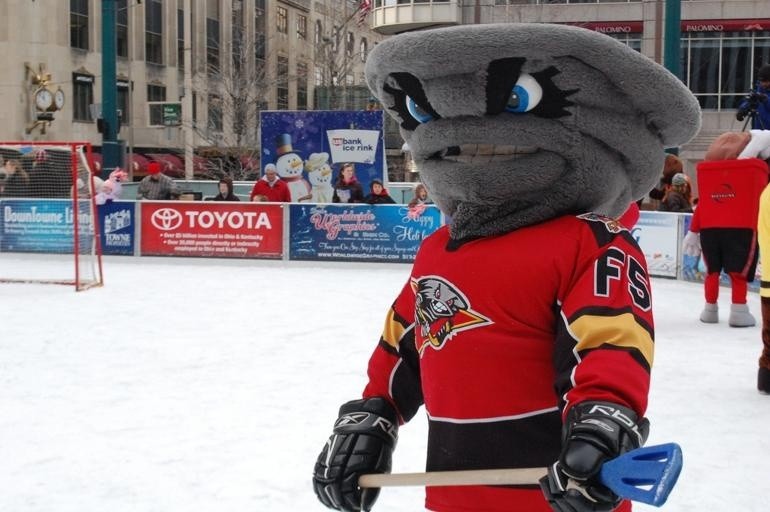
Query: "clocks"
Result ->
[[24, 61, 64, 134]]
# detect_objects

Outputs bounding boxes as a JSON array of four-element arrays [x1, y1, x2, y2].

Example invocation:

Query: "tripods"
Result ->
[[742, 110, 764, 131]]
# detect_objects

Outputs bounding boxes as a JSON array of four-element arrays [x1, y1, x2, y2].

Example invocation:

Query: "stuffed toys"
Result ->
[[94, 167, 128, 206], [650, 154, 683, 201], [309, 20, 701, 512], [681, 129, 770, 329]]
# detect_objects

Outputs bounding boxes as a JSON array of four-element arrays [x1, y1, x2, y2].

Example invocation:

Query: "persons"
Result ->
[[213, 177, 240, 202], [755, 182, 770, 394], [736, 64, 769, 130], [252, 195, 267, 203], [0, 148, 105, 204], [249, 163, 292, 203], [332, 163, 364, 204], [364, 180, 397, 204], [409, 185, 435, 208], [660, 174, 694, 214], [135, 163, 178, 200]]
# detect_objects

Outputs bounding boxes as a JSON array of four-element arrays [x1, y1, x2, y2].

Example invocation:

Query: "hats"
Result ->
[[147, 163, 161, 175], [303, 152, 329, 172], [102, 179, 114, 189], [35, 150, 49, 161], [672, 173, 688, 186], [273, 133, 303, 162]]
